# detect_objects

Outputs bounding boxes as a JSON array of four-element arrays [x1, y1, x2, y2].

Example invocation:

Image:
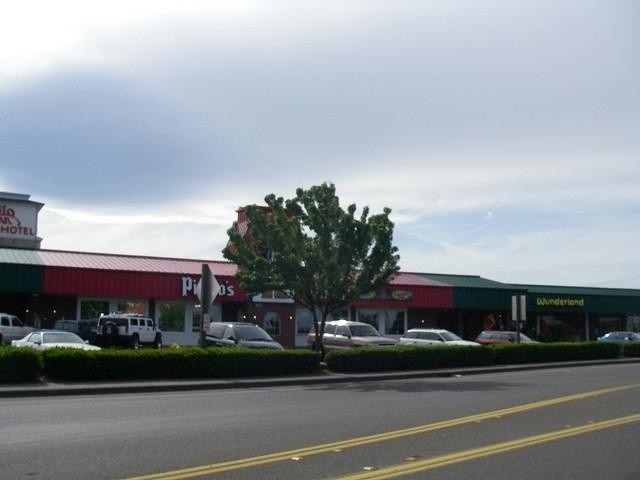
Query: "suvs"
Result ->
[[96, 311, 163, 350]]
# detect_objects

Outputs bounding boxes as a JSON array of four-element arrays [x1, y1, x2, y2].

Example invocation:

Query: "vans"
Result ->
[[197, 322, 285, 349]]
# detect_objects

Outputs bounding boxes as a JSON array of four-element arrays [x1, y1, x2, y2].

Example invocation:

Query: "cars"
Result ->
[[475, 331, 540, 344], [597, 331, 640, 341], [399, 328, 482, 347], [11, 329, 102, 351], [0, 313, 37, 345], [306, 319, 399, 352]]
